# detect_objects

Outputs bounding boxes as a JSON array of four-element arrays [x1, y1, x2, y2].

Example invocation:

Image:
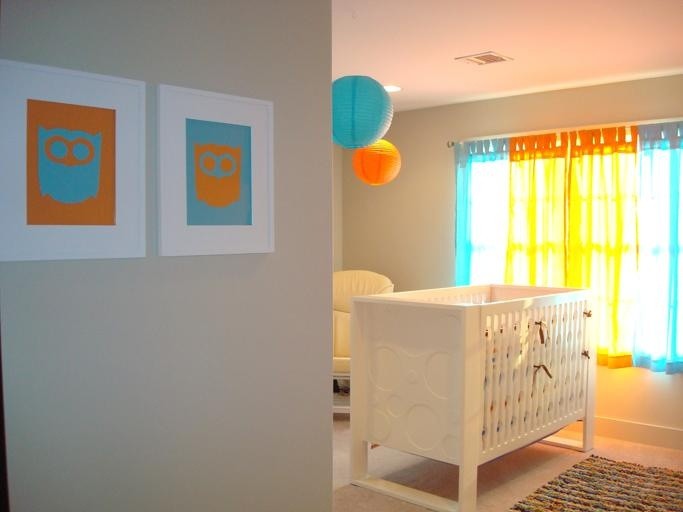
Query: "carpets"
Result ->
[[506, 454, 683, 512]]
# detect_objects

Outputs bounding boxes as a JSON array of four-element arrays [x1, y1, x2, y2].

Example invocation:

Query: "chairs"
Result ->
[[333, 269, 394, 414]]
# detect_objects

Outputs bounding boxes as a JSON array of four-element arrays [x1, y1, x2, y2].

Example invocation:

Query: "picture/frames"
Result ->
[[0, 58, 146, 263], [156, 83, 274, 257]]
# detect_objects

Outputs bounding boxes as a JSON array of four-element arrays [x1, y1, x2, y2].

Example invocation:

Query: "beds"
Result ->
[[350, 282, 598, 512]]
